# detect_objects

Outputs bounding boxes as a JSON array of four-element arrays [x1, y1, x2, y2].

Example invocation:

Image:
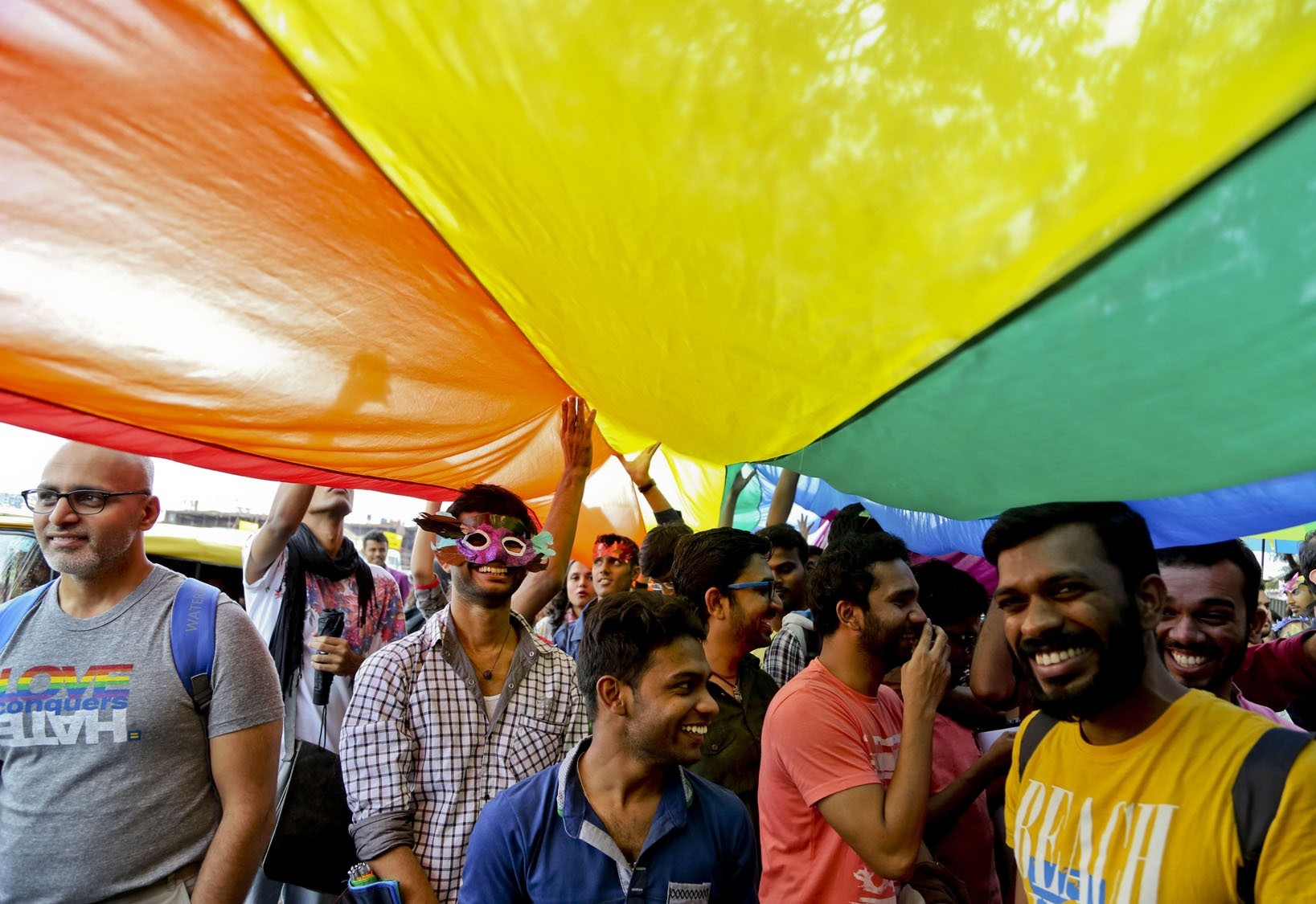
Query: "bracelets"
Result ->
[[638, 478, 657, 494]]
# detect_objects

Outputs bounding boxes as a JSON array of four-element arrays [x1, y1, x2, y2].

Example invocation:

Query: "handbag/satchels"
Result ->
[[260, 741, 362, 896]]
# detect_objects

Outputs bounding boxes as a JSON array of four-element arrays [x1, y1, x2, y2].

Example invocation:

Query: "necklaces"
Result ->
[[710, 671, 745, 704], [463, 625, 513, 679]]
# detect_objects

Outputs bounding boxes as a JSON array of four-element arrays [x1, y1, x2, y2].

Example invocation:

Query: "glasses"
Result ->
[[22, 487, 150, 516], [721, 581, 777, 601]]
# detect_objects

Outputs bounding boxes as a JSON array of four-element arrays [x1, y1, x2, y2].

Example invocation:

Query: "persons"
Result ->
[[1, 330, 1316, 903]]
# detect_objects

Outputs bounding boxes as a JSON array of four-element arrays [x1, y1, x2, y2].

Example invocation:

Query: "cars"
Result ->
[[0, 515, 263, 629]]
